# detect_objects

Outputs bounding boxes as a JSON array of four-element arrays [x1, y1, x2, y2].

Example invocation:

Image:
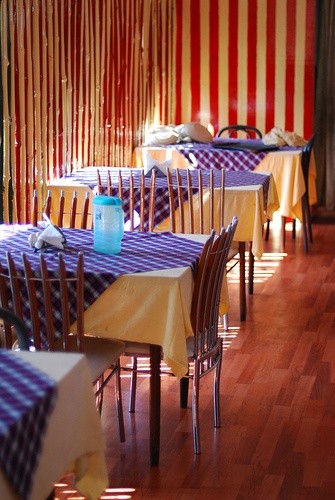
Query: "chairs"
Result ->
[[2, 250, 126, 442], [96, 216, 238, 455], [30, 189, 99, 413], [217, 126, 262, 140], [96, 168, 228, 330], [265, 134, 317, 252]]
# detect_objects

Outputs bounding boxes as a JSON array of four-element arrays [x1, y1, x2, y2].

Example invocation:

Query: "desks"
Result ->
[[47, 167, 280, 321], [1, 350, 109, 500], [132, 140, 306, 254], [1, 224, 220, 412]]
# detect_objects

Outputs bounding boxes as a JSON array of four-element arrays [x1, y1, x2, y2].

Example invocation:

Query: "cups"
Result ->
[[93, 195, 123, 255]]
